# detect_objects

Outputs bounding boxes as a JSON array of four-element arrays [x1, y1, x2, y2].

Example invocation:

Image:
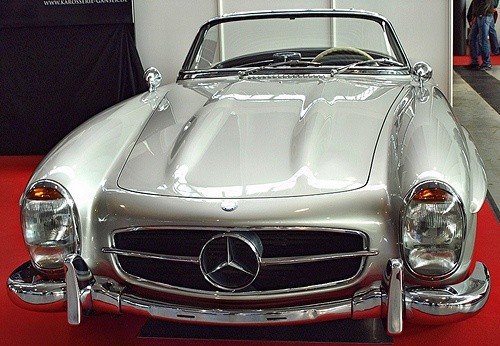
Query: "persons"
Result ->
[[467, 0, 500, 70]]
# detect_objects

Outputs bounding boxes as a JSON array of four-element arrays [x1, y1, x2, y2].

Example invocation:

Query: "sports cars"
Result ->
[[6, 7, 491, 344]]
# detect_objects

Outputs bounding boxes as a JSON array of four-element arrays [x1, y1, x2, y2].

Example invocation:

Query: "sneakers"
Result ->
[[465, 63, 480, 69], [479, 64, 492, 71]]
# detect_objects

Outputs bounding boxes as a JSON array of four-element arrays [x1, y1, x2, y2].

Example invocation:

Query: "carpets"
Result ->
[[453, 53, 500, 66], [0, 151, 500, 346]]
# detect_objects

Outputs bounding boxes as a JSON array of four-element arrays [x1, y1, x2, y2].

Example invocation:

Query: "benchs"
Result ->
[[213, 47, 395, 72]]
